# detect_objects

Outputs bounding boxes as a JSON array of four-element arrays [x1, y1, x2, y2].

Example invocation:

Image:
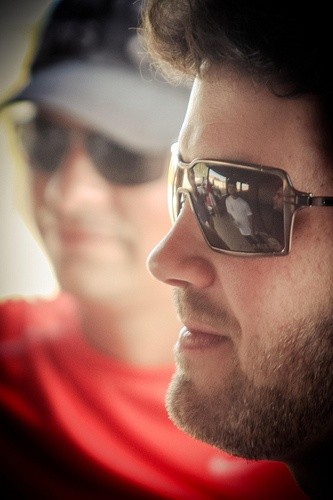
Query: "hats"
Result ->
[[0, 0, 196, 154]]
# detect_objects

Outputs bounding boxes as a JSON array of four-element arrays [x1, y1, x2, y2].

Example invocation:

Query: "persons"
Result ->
[[0, 0, 309, 500], [201, 176, 223, 217], [252, 186, 285, 252], [224, 180, 271, 252], [135, 0, 333, 500]]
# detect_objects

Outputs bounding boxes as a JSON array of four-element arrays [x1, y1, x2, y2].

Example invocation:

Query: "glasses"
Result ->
[[167, 142, 333, 257], [15, 113, 165, 185]]
